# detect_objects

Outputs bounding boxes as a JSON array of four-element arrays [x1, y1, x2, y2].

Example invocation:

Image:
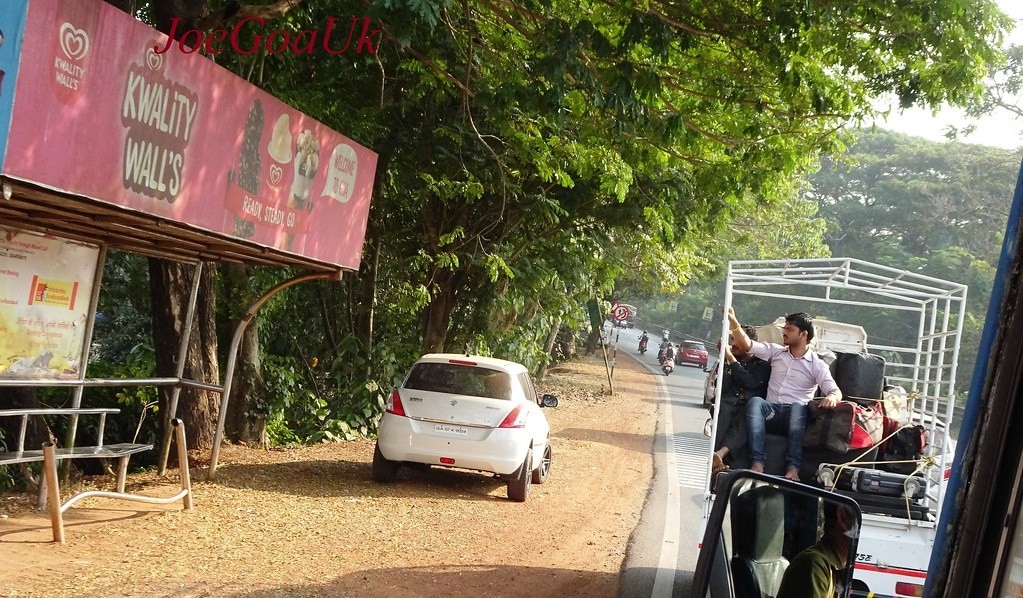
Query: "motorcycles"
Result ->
[[637, 334, 649, 354]]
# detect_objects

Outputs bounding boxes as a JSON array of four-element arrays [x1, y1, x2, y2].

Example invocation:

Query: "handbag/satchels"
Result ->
[[802, 399, 883, 455], [881, 416, 926, 454]]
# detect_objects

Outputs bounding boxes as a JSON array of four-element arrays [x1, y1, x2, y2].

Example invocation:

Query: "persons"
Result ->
[[637, 329, 649, 352], [721, 304, 844, 485], [663, 341, 676, 361], [709, 323, 771, 483], [663, 330, 670, 334], [657, 338, 668, 358]]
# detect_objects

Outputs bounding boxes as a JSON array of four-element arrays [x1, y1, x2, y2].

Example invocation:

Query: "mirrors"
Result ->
[[689, 470, 866, 598]]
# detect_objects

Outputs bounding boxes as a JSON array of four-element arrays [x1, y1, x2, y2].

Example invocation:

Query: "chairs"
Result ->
[[729, 485, 793, 597]]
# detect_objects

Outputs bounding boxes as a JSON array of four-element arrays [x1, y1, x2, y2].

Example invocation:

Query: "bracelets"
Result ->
[[730, 324, 741, 331]]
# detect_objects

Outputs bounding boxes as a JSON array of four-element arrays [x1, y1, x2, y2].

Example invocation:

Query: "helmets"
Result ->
[[668, 342, 673, 346], [644, 330, 647, 333]]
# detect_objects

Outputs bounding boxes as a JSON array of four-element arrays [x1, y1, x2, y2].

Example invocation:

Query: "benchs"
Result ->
[[0, 406, 156, 512]]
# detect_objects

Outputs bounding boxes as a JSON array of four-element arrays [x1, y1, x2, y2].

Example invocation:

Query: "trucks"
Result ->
[[706, 254, 971, 598]]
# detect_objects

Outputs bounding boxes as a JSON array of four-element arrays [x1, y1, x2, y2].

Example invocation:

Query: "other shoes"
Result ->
[[645, 349, 647, 351], [638, 349, 640, 351], [657, 357, 659, 359]]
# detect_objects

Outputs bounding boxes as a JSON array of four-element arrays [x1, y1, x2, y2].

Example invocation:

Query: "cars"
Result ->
[[675, 340, 708, 368], [370, 350, 559, 503]]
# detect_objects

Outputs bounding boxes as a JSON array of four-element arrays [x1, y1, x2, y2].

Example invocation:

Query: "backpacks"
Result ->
[[667, 348, 673, 358], [641, 334, 648, 342]]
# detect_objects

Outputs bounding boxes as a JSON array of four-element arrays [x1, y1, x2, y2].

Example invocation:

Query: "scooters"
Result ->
[[657, 344, 674, 375]]
